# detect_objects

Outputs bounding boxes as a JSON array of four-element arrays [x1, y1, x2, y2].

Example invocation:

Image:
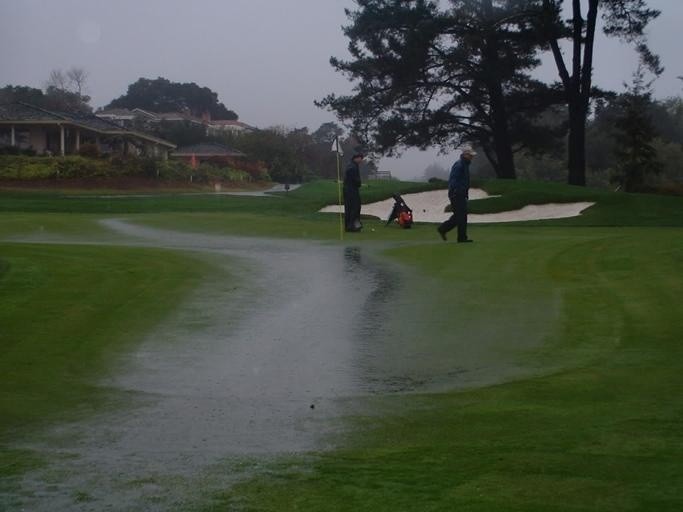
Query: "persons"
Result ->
[[343, 152, 364, 232], [437, 145, 478, 243]]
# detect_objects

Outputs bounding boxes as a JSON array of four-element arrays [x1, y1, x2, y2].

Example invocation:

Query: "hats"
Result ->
[[463, 146, 477, 156]]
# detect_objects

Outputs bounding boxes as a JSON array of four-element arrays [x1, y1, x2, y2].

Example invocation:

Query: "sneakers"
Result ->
[[457, 239, 473, 242], [438, 228, 447, 241]]
